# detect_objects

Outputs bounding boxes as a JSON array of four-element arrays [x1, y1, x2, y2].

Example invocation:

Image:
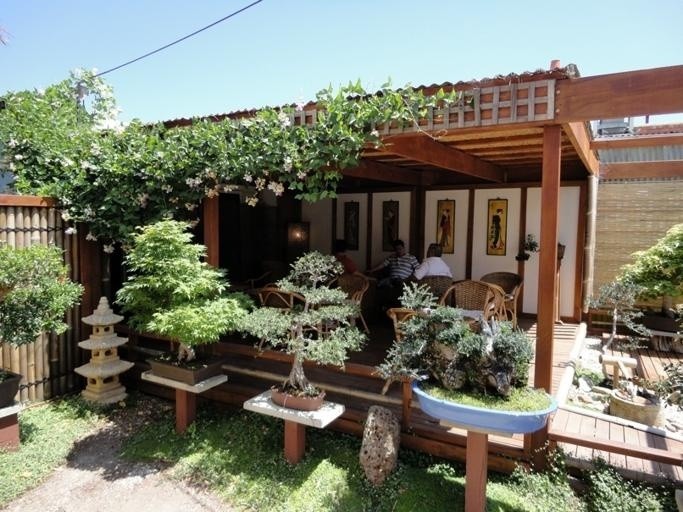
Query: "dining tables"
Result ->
[[114, 217, 255, 386], [563, 356, 683, 427], [235, 249, 370, 411], [613, 221, 682, 333], [0, 240, 90, 409], [374, 277, 559, 431]]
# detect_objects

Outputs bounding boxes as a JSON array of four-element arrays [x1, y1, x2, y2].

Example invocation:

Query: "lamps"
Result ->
[[251, 268, 525, 394]]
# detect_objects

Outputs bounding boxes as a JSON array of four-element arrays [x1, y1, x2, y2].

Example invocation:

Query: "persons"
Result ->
[[332, 240, 369, 280], [490, 209, 504, 248], [413, 244, 452, 280], [439, 209, 450, 247], [387, 210, 395, 244], [366, 240, 420, 288]]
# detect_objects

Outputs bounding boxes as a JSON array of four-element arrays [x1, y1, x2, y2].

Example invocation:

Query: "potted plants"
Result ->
[[343, 200, 359, 251], [435, 197, 455, 255], [381, 200, 400, 253], [486, 196, 508, 256]]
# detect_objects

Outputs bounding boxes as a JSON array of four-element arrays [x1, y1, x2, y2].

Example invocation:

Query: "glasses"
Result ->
[[286, 220, 311, 254]]
